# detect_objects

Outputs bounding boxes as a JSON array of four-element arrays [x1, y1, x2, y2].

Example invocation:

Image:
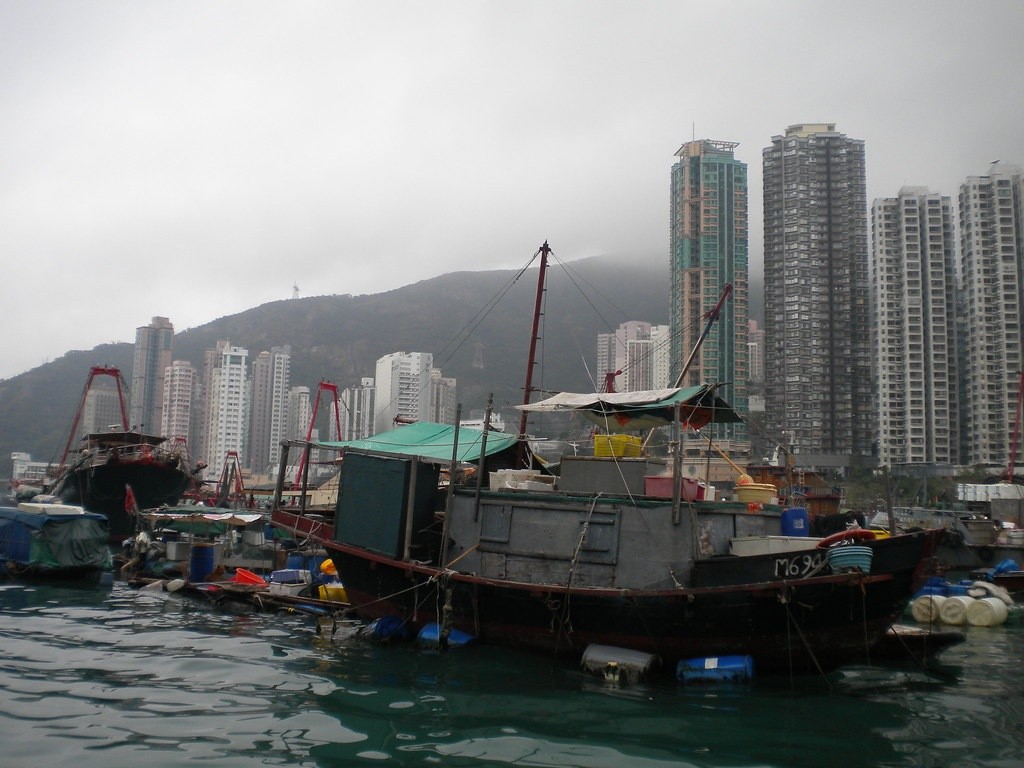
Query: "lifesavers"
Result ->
[[816, 529, 876, 549]]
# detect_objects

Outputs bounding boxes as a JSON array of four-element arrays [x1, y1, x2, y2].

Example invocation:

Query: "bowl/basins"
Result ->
[[235, 568, 265, 585], [318, 586, 347, 603], [826, 546, 874, 575], [733, 483, 777, 504]]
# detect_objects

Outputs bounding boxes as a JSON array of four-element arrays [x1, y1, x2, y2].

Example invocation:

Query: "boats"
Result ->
[[0, 240, 1023, 692]]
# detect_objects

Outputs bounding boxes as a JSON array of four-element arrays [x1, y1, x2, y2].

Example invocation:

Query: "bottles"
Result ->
[[167, 579, 185, 592]]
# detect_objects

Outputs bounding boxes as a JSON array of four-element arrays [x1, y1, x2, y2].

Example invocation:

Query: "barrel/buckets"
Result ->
[[188, 545, 214, 582], [676, 654, 756, 687], [967, 521, 993, 544], [361, 615, 411, 642], [911, 595, 947, 623], [308, 555, 331, 586], [967, 597, 1008, 627], [939, 595, 976, 625], [580, 643, 663, 688], [416, 622, 476, 647], [99, 572, 113, 586], [30, 495, 63, 505], [781, 508, 809, 537], [286, 553, 305, 570]]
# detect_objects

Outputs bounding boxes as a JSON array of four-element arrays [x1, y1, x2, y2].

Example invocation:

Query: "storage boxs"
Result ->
[[489, 472, 557, 492], [594, 433, 644, 459], [165, 529, 349, 604], [643, 474, 716, 503]]
